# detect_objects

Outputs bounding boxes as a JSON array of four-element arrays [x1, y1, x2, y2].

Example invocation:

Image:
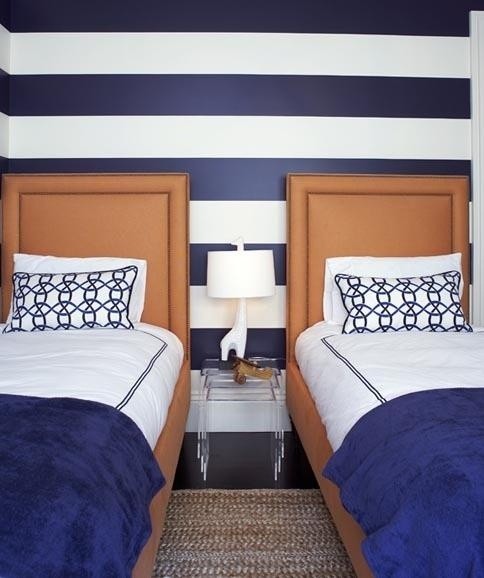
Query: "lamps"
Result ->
[[204, 237, 276, 368]]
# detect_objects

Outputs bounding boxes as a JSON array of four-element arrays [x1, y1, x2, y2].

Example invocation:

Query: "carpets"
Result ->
[[152, 488, 359, 578]]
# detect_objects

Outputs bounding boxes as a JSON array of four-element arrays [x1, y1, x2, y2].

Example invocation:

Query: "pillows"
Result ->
[[10, 250, 147, 332], [322, 252, 473, 334]]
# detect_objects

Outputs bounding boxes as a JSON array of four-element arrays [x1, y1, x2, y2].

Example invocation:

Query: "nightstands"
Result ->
[[181, 367, 297, 489]]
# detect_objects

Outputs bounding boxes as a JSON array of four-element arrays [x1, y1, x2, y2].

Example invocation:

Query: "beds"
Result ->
[[0, 172, 192, 578], [285, 174, 484, 578]]
